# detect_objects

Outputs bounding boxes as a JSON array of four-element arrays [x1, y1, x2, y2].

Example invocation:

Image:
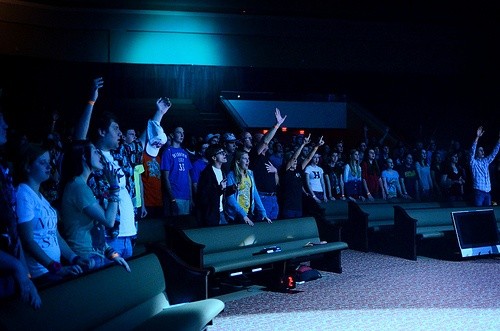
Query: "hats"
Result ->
[[207, 131, 220, 139], [145, 118, 168, 157]]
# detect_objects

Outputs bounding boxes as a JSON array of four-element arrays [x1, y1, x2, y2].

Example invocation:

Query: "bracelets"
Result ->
[[89, 100, 95, 105], [112, 253, 118, 258], [72, 256, 81, 265], [69, 254, 77, 262], [108, 187, 120, 192], [107, 194, 120, 202], [105, 247, 115, 257], [47, 262, 61, 272]]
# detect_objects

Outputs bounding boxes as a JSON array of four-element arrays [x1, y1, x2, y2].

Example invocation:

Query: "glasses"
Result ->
[[213, 150, 227, 157]]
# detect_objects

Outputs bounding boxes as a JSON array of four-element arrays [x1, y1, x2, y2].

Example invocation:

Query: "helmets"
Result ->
[[239, 131, 252, 139], [219, 132, 236, 141]]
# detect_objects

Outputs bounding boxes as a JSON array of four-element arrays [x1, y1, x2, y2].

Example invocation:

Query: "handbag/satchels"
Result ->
[[293, 264, 322, 282], [250, 268, 296, 292]]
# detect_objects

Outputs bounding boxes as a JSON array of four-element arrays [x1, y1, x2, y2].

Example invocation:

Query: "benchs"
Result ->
[[0, 252, 225, 331], [393, 205, 500, 261], [347, 200, 473, 252], [307, 195, 427, 241], [182, 216, 349, 277]]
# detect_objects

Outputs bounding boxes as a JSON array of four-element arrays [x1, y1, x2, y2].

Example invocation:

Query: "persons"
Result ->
[[469, 127, 500, 206], [0, 78, 500, 308]]
